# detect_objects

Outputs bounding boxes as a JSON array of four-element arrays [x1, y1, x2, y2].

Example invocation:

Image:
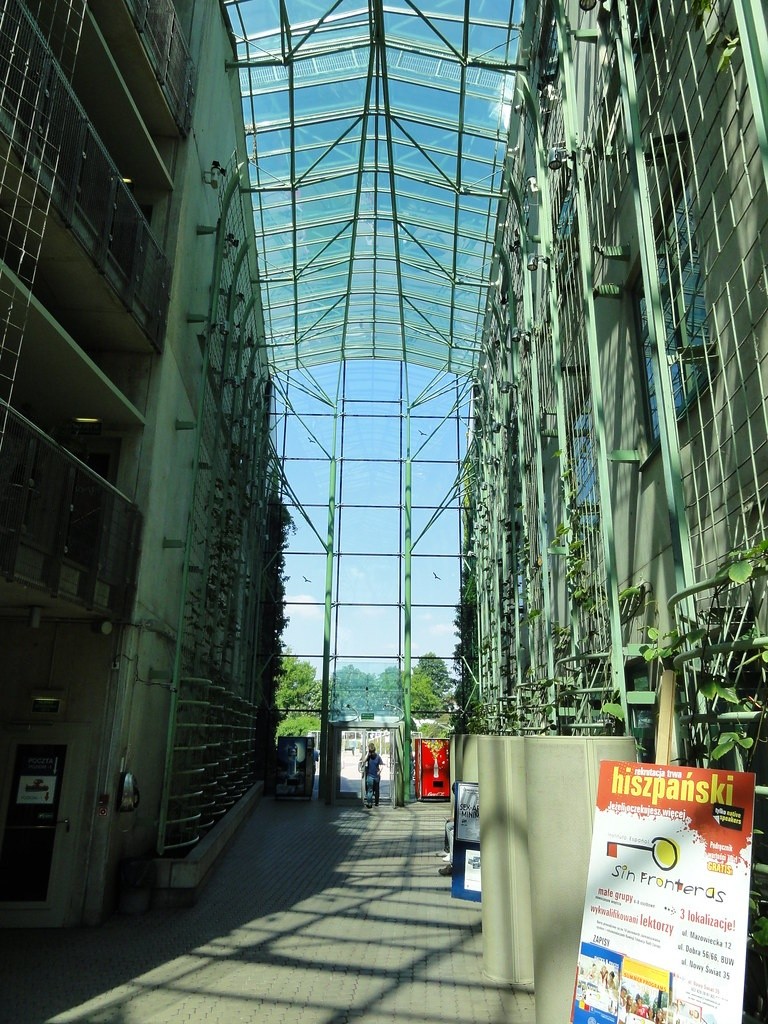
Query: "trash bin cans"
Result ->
[[117, 858, 155, 915]]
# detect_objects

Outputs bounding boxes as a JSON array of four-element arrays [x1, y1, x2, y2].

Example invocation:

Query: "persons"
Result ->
[[358, 743, 383, 808], [436, 782, 455, 876], [410, 738, 415, 780], [311, 744, 319, 791], [579, 964, 675, 1024]]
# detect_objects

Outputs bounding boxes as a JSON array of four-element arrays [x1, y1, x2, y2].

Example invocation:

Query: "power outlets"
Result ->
[[111, 662, 119, 671]]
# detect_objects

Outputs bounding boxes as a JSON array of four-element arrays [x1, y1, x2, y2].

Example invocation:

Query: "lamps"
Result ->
[[501, 381, 509, 393], [527, 253, 538, 271], [219, 320, 230, 335], [476, 505, 482, 511], [549, 147, 563, 169], [486, 455, 493, 464], [247, 452, 253, 462], [474, 522, 479, 529], [261, 518, 267, 526], [240, 416, 248, 428], [481, 482, 488, 490], [232, 375, 240, 388], [579, 0, 597, 11], [96, 621, 113, 635], [470, 537, 476, 544], [510, 327, 522, 342], [466, 551, 472, 557], [255, 500, 263, 508], [264, 534, 269, 542], [493, 423, 501, 433]]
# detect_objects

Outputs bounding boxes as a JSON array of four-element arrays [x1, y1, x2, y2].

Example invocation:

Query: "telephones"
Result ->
[[115, 768, 140, 814]]
